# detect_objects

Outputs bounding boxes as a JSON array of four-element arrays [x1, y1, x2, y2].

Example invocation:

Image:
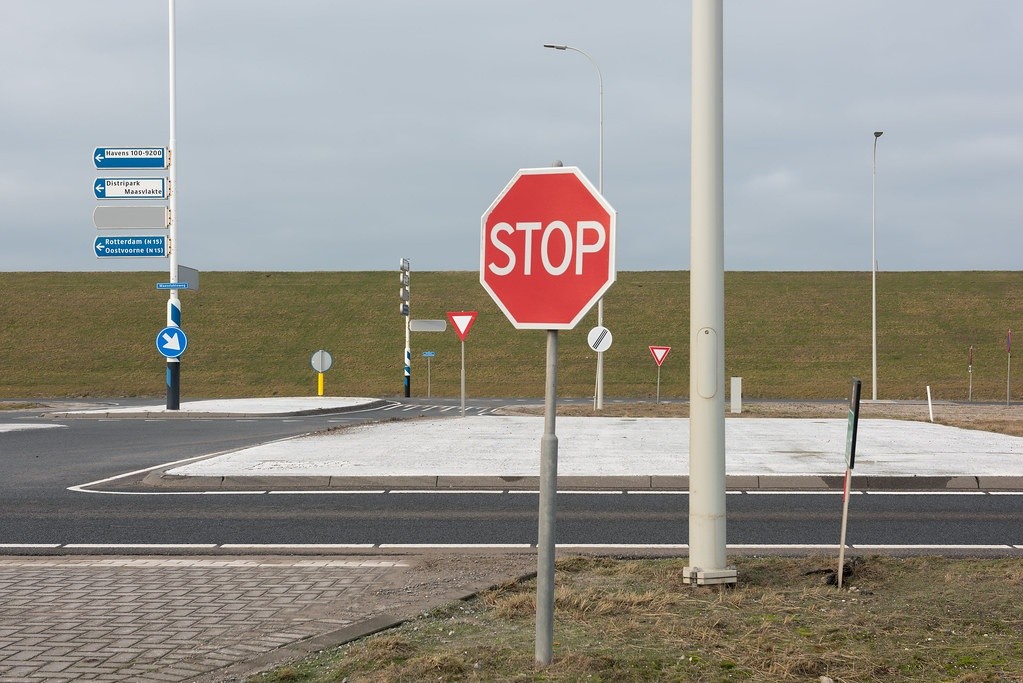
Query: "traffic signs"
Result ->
[[92, 147, 168, 171], [92, 234, 169, 258], [92, 176, 169, 202]]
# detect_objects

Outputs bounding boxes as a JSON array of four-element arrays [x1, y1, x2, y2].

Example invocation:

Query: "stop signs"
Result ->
[[479, 165, 618, 330]]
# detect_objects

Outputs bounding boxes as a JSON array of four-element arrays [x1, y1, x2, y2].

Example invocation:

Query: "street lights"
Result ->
[[871, 131, 883, 401], [543, 44, 607, 411]]
